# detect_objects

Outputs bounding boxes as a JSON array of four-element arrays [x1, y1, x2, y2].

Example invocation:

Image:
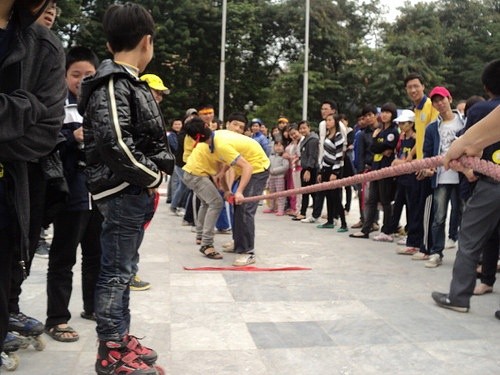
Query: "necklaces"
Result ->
[[2, 12, 13, 28]]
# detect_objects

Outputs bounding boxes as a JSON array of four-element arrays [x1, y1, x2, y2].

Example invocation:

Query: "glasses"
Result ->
[[44, 3, 62, 17]]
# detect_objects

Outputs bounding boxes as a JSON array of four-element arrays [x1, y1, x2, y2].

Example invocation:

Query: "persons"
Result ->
[[42, 45, 104, 342], [129, 74, 171, 291], [182, 111, 246, 259], [166, 73, 500, 312], [27, 0, 71, 278], [0, 0, 69, 371], [78, 3, 177, 375], [184, 117, 271, 266], [443, 105, 500, 171]]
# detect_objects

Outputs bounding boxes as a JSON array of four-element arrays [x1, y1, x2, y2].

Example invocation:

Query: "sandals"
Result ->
[[200, 245, 222, 259], [196, 238, 202, 244], [46, 325, 79, 341], [81, 311, 98, 320]]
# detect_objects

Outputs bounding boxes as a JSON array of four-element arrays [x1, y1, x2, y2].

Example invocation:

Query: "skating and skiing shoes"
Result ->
[[0, 334, 22, 371], [7, 311, 46, 352]]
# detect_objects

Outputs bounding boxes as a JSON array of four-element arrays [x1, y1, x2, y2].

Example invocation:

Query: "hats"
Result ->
[[139, 74, 168, 90], [393, 110, 416, 123], [249, 118, 261, 130], [186, 108, 197, 116], [430, 87, 450, 99]]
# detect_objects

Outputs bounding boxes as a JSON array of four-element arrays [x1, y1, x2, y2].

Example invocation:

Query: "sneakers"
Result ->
[[128, 275, 149, 290], [121, 332, 158, 365], [432, 292, 468, 312], [95, 341, 164, 375], [472, 284, 493, 295]]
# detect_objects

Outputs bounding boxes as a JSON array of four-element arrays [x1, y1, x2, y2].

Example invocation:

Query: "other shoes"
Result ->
[[213, 229, 229, 234], [265, 207, 459, 268], [223, 246, 236, 253], [35, 245, 50, 258], [233, 254, 255, 266], [182, 219, 193, 226], [191, 226, 196, 232]]
